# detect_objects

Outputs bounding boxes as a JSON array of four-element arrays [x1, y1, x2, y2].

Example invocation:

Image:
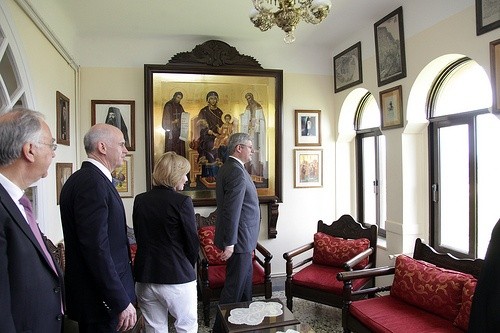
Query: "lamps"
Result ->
[[248, 0, 332, 45]]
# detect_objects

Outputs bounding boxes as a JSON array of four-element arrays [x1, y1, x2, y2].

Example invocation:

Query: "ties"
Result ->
[[18, 194, 57, 275]]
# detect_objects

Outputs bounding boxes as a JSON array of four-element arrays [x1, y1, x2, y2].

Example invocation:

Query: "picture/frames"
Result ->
[[379, 85, 404, 131], [333, 41, 363, 94], [56, 91, 71, 146], [295, 110, 321, 146], [475, 0, 500, 36], [143, 63, 283, 203], [293, 149, 323, 188], [55, 163, 73, 205], [111, 154, 134, 198], [91, 100, 135, 151], [373, 7, 407, 87]]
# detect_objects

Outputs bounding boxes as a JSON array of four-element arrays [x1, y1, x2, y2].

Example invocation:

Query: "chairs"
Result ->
[[195, 209, 272, 327], [336, 238, 484, 333], [283, 215, 378, 313]]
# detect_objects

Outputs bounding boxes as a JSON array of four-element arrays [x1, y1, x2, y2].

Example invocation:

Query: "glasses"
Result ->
[[39, 143, 58, 152], [235, 144, 253, 151]]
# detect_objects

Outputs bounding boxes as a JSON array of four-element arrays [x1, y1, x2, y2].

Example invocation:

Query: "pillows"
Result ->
[[197, 226, 255, 265], [390, 254, 477, 328], [313, 233, 370, 270]]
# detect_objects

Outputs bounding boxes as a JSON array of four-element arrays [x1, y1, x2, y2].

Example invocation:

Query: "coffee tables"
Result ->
[[214, 298, 316, 333]]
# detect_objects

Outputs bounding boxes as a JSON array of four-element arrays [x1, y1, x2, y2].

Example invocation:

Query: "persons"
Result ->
[[60, 124, 137, 333], [132, 152, 198, 333], [0, 108, 68, 333], [213, 133, 261, 304]]
